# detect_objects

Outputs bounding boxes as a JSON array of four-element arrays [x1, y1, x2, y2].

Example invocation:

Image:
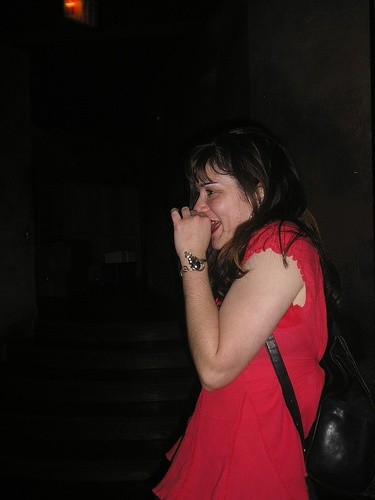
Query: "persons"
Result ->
[[142, 126, 333, 500]]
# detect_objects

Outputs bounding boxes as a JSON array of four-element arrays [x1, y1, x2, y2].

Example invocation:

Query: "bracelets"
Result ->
[[179, 252, 208, 278]]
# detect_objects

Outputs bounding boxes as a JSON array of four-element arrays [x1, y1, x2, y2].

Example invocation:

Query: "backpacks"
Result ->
[[265, 221, 375, 490]]
